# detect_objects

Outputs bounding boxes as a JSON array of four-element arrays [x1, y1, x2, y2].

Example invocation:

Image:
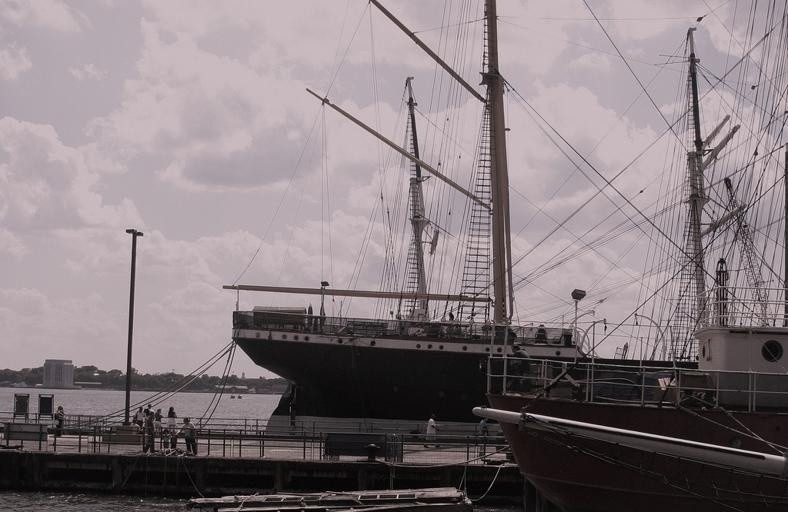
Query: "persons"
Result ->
[[54, 406, 65, 434], [134, 403, 196, 456], [479, 417, 489, 457], [424, 413, 440, 448]]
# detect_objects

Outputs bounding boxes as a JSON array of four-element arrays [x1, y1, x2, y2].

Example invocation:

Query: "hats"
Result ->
[[137, 403, 154, 408]]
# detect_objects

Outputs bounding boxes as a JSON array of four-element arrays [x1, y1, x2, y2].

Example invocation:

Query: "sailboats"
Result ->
[[220, 0, 788, 512]]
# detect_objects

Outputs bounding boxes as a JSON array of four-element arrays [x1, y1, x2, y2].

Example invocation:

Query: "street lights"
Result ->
[[119, 228, 146, 427]]
